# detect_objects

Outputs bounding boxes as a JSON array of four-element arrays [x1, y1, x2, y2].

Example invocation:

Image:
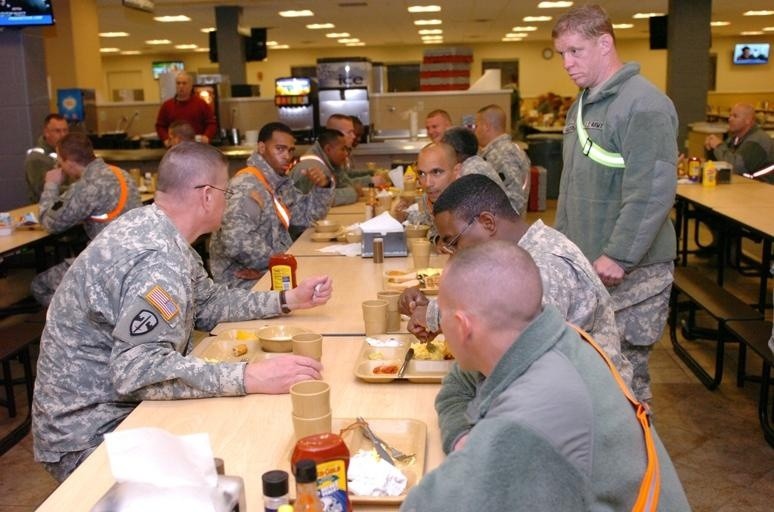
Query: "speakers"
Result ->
[[250, 26, 267, 59], [648, 14, 669, 50], [208, 30, 218, 61]]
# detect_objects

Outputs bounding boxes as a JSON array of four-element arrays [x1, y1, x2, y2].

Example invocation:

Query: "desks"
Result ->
[[33, 169, 445, 512]]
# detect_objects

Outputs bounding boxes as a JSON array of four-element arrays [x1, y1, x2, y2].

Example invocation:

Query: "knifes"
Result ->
[[360, 422, 394, 469]]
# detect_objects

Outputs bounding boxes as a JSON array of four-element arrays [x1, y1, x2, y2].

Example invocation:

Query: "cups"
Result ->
[[373, 238, 384, 264], [411, 241, 431, 269], [362, 290, 403, 335], [290, 333, 333, 436]]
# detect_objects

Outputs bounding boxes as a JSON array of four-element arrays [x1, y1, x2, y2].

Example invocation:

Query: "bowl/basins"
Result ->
[[312, 218, 341, 232], [255, 325, 311, 353], [405, 224, 431, 238]]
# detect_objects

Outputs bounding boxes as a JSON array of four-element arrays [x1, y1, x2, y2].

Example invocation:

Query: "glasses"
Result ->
[[440, 215, 479, 255], [194, 184, 235, 200]]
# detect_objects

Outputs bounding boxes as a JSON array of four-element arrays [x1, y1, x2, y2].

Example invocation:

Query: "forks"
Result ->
[[382, 442, 417, 462]]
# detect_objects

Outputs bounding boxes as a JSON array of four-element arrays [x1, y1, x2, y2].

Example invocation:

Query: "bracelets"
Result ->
[[279, 290, 291, 314]]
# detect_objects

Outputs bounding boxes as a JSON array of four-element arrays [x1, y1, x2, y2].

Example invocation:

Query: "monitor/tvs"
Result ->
[[151, 60, 184, 81], [0, 0, 56, 27], [732, 41, 771, 64]]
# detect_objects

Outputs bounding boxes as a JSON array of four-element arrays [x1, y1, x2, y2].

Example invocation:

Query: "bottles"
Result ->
[[210, 457, 323, 512], [366, 183, 375, 217]]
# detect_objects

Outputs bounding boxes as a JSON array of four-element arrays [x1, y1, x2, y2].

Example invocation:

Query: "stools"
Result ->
[[2, 322, 41, 444]]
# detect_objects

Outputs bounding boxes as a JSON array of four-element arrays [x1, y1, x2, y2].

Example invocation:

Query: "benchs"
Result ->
[[726, 320, 774, 452], [668, 266, 763, 390]]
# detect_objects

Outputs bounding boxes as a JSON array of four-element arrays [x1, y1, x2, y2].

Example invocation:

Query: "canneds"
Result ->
[[688, 157, 701, 182]]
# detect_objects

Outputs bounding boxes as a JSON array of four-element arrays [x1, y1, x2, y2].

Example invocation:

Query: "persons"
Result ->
[[694, 102, 774, 268], [31, 143, 332, 485], [547, 0, 680, 408], [400, 238, 693, 512], [736, 46, 752, 60], [154, 71, 219, 277], [396, 174, 634, 388], [753, 48, 758, 57], [209, 114, 394, 296], [29, 132, 144, 309], [23, 113, 71, 202], [168, 121, 196, 146], [395, 104, 532, 254]]
